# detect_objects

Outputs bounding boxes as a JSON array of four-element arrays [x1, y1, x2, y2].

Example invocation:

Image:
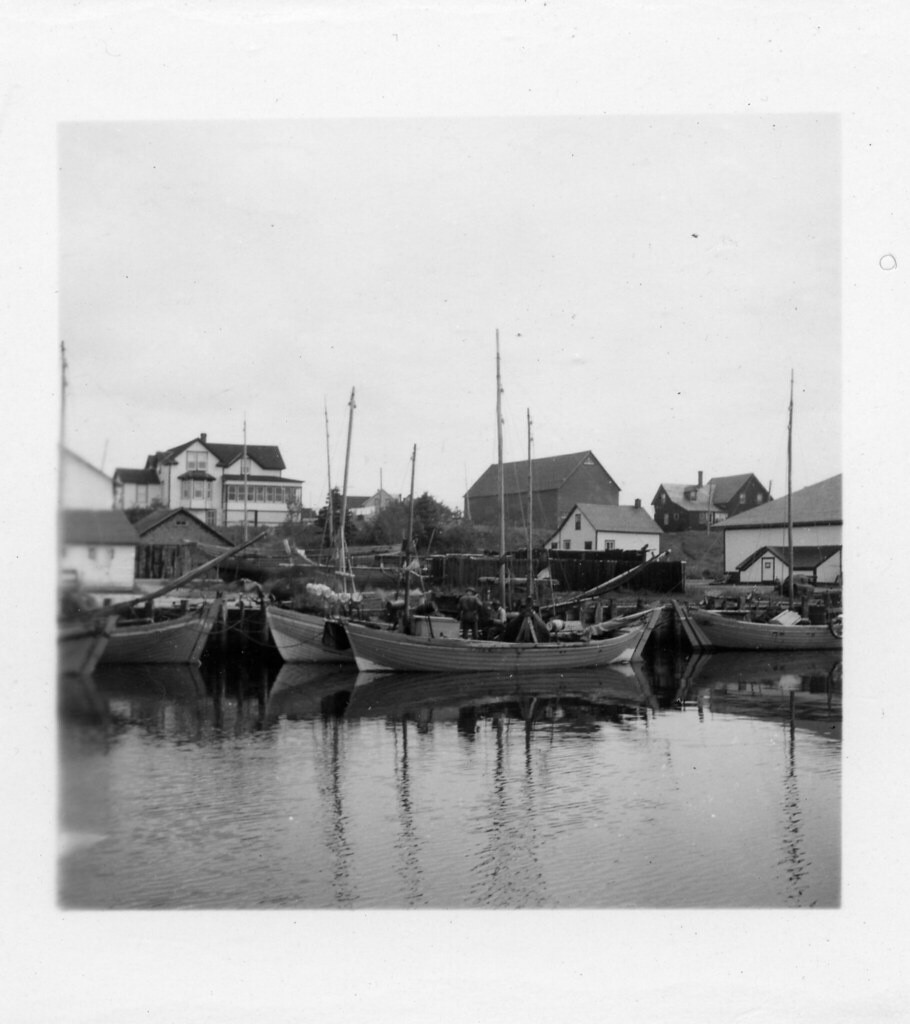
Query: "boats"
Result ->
[[671, 647, 841, 840], [59, 381, 843, 679], [259, 660, 660, 814], [686, 364, 843, 652], [342, 326, 674, 675]]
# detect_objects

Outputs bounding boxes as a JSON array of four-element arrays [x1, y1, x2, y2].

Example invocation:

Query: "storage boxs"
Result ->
[[410, 614, 460, 638]]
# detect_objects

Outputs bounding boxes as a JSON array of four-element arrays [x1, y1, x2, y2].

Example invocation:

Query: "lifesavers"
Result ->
[[830, 616, 843, 639]]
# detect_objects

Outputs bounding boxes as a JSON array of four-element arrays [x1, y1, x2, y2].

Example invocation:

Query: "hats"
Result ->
[[466, 587, 476, 594], [491, 600, 501, 605]]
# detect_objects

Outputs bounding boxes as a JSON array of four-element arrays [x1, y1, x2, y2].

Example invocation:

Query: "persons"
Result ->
[[459, 586, 484, 640], [348, 599, 360, 617], [486, 600, 507, 642], [331, 600, 347, 617], [742, 606, 757, 622]]
